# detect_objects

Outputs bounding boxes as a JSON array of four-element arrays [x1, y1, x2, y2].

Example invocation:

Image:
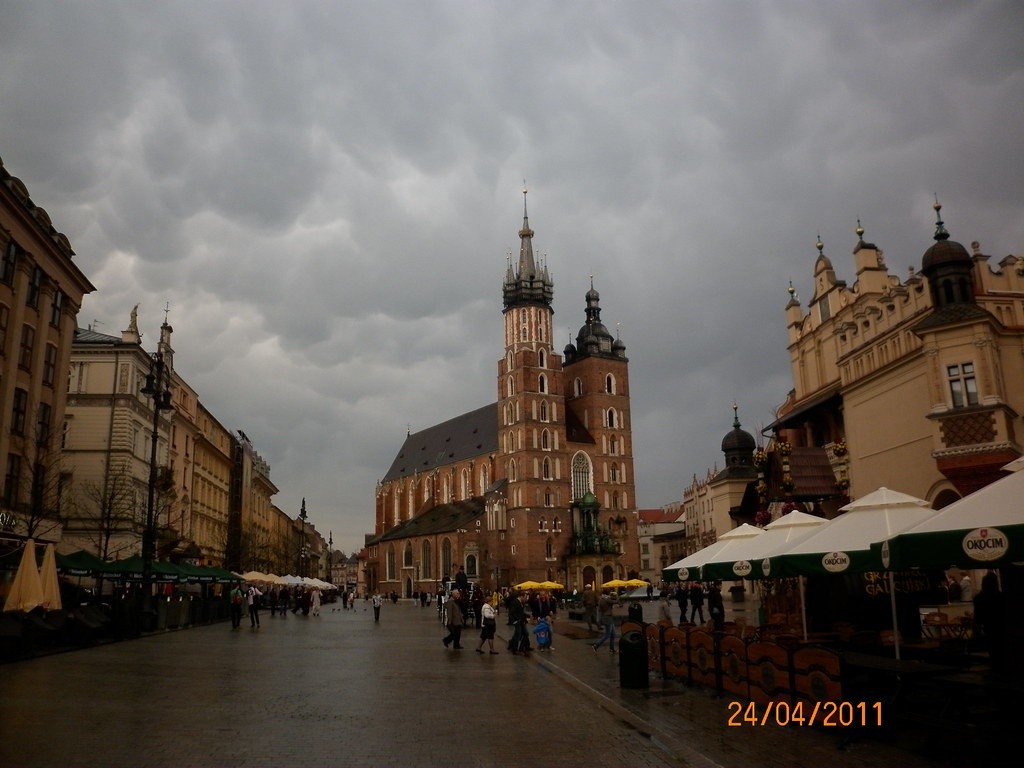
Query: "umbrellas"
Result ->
[[540, 580, 564, 594], [39, 541, 63, 611], [0, 546, 338, 598], [513, 580, 540, 591], [2, 539, 43, 614], [699, 510, 830, 642], [662, 523, 768, 627], [868, 455, 1024, 573], [757, 487, 937, 680], [600, 579, 628, 592], [626, 578, 650, 586]]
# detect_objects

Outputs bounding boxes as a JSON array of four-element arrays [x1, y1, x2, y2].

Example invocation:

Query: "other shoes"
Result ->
[[317, 613, 319, 616], [251, 623, 255, 628], [701, 620, 706, 623], [513, 651, 521, 655], [608, 649, 618, 654], [592, 645, 599, 653], [588, 628, 592, 632], [313, 613, 315, 616], [257, 624, 260, 629], [476, 648, 485, 654], [490, 650, 499, 654], [525, 647, 534, 652], [442, 639, 449, 648], [453, 646, 463, 649], [598, 628, 604, 630]]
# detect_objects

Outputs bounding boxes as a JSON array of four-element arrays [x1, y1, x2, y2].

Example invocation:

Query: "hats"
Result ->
[[459, 566, 465, 569]]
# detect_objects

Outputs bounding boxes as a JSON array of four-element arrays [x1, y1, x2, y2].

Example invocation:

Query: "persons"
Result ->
[[228, 583, 399, 632], [441, 589, 464, 649], [475, 596, 499, 654], [413, 565, 623, 657], [707, 580, 726, 631], [689, 582, 706, 627], [646, 580, 713, 599], [803, 570, 1024, 748], [674, 582, 690, 623]]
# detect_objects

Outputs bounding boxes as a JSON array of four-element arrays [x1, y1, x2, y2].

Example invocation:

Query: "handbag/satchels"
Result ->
[[482, 608, 496, 626], [233, 589, 243, 606], [247, 590, 253, 605]]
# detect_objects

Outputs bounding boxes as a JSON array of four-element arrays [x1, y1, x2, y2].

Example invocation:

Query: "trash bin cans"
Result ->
[[619, 629, 649, 692]]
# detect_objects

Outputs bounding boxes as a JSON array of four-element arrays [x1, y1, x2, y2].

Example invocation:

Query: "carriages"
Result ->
[[441, 581, 475, 626]]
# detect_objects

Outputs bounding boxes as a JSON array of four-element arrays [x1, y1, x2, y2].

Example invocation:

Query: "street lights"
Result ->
[[299, 497, 308, 577], [141, 352, 176, 592]]
